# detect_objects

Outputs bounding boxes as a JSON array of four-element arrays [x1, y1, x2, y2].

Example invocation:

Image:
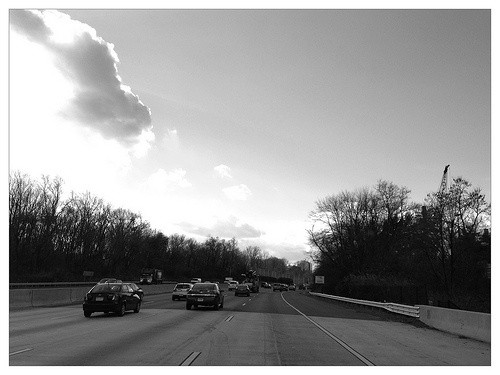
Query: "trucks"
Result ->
[[224, 277, 233, 283], [139, 270, 163, 285], [191, 277, 201, 284]]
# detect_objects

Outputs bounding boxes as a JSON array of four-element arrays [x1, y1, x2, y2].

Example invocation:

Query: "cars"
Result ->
[[273, 283, 306, 292], [172, 283, 194, 301], [261, 282, 267, 287], [113, 282, 144, 302], [228, 281, 239, 291], [186, 283, 224, 310], [234, 284, 250, 297], [83, 283, 142, 317], [265, 284, 271, 288]]
